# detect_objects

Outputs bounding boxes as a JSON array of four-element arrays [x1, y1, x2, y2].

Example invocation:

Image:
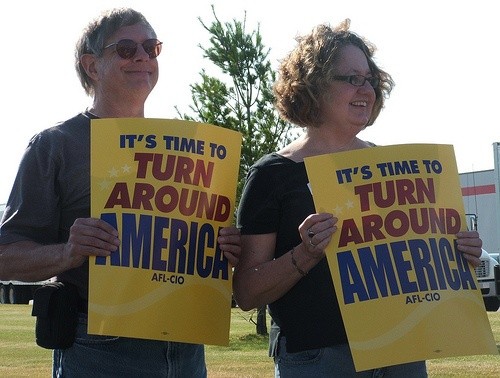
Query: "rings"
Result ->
[[309, 241, 315, 247], [308, 229, 315, 237]]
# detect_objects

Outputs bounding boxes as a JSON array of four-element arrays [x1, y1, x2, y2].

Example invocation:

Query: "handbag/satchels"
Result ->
[[31, 282, 79, 350]]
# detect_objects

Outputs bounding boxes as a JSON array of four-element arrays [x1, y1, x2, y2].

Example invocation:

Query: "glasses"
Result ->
[[95, 37, 163, 61], [331, 72, 382, 87]]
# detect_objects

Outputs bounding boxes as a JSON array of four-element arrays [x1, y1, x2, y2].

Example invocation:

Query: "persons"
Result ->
[[0, 7, 241, 378], [233, 16, 482, 378]]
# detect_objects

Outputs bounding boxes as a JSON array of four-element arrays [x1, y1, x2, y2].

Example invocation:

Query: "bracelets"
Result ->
[[291, 247, 305, 279]]
